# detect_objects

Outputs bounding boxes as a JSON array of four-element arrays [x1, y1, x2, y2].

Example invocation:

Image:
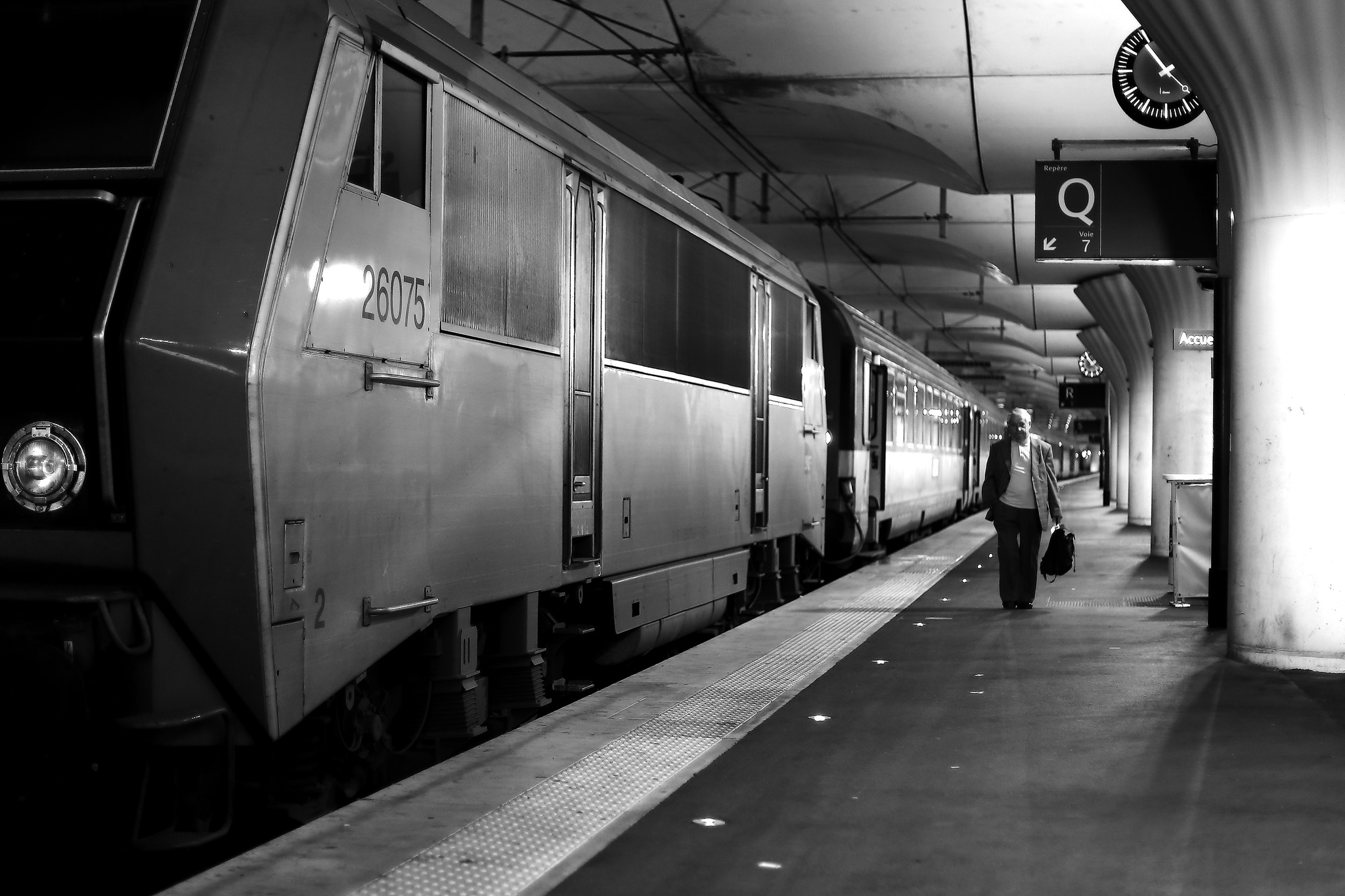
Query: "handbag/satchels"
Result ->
[[1040, 523, 1076, 583]]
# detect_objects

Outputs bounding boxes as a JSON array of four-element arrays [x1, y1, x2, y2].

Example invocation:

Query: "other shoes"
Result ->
[[1002, 601, 1017, 609], [1017, 602, 1033, 609]]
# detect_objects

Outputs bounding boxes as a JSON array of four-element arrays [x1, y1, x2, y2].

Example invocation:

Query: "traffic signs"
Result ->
[[1075, 420, 1101, 434], [1035, 160, 1218, 261], [1059, 383, 1106, 409]]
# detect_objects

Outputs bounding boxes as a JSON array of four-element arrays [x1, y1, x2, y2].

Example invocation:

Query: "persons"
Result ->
[[982, 407, 1063, 610]]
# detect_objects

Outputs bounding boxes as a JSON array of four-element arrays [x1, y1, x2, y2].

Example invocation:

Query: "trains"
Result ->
[[0, 0, 1092, 840]]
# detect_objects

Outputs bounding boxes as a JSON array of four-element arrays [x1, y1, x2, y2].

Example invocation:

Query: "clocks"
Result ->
[[1111, 26, 1206, 129], [1078, 350, 1103, 378]]
